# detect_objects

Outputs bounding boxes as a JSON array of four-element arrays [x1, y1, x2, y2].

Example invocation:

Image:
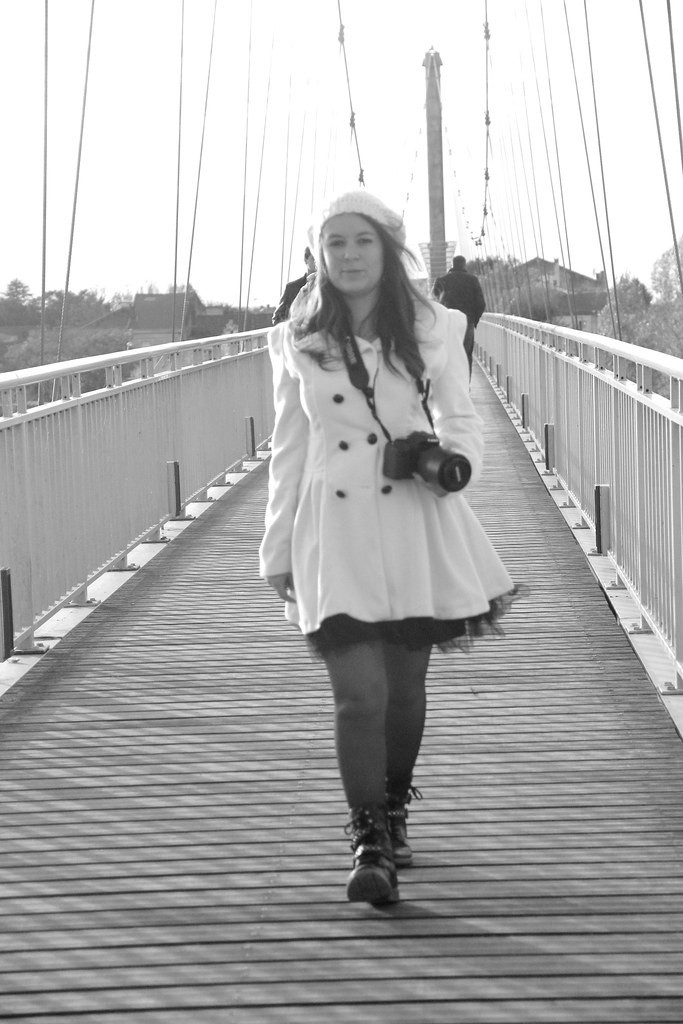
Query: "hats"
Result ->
[[308, 191, 405, 260]]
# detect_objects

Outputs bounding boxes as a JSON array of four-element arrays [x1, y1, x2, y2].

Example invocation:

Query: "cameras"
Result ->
[[381, 430, 473, 496]]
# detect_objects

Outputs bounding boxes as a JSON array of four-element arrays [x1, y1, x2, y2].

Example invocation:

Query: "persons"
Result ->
[[271, 245, 316, 327], [432, 254, 485, 386], [260, 192, 519, 911]]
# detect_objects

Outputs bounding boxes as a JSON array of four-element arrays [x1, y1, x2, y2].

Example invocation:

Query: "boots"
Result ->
[[390, 807, 414, 865], [344, 804, 401, 906]]
